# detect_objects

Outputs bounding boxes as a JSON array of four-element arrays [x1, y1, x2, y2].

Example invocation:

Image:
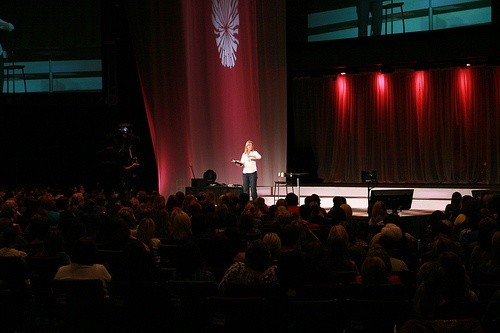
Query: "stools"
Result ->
[[274, 181, 287, 207]]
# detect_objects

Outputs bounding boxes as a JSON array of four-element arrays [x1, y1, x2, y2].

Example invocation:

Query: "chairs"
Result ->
[[1, 197, 499, 333]]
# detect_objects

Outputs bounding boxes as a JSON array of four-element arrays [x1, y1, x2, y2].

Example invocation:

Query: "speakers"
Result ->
[[185, 186, 242, 198]]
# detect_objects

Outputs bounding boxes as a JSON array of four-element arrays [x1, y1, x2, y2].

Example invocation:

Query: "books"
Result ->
[[232, 159, 244, 166]]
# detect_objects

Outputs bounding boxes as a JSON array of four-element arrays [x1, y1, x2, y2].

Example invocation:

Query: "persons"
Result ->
[[0, 180, 500, 333], [123, 142, 143, 188], [235, 139, 262, 200]]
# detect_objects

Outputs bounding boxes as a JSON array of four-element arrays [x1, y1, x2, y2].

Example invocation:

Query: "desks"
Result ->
[[184, 186, 242, 203], [278, 172, 308, 205]]
